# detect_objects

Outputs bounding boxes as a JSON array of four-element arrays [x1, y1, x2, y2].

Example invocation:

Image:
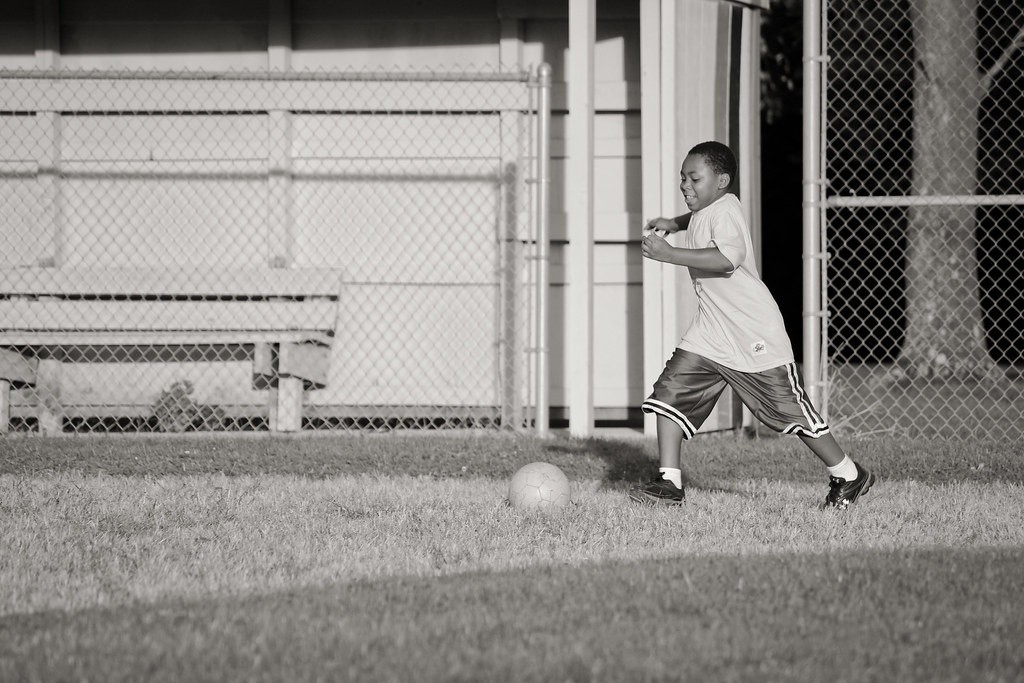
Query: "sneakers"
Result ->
[[629, 471, 686, 507], [821, 460, 875, 511]]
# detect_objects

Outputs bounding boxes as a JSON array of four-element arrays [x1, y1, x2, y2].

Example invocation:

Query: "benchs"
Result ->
[[0, 269, 344, 433]]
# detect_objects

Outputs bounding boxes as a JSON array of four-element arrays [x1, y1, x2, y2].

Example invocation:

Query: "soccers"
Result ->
[[508, 461, 569, 516]]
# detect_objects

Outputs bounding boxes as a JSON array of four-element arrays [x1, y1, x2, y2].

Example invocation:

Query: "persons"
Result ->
[[628, 140, 877, 508]]
